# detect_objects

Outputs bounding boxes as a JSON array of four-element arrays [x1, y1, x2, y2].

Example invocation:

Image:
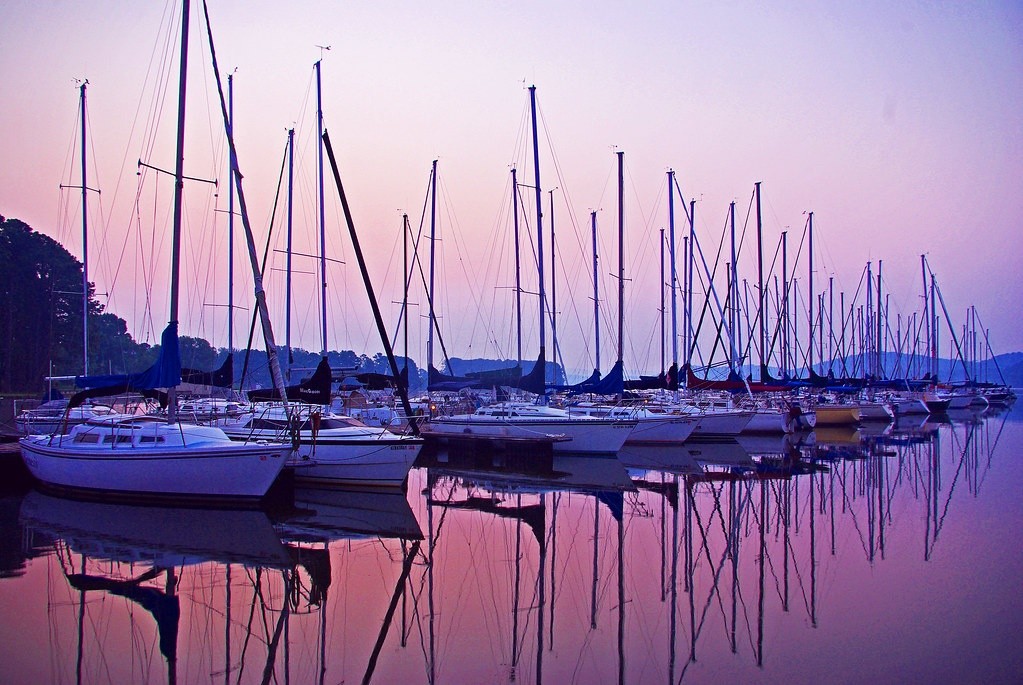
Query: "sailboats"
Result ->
[[17, 0, 1019, 506], [14, 415, 982, 685]]
